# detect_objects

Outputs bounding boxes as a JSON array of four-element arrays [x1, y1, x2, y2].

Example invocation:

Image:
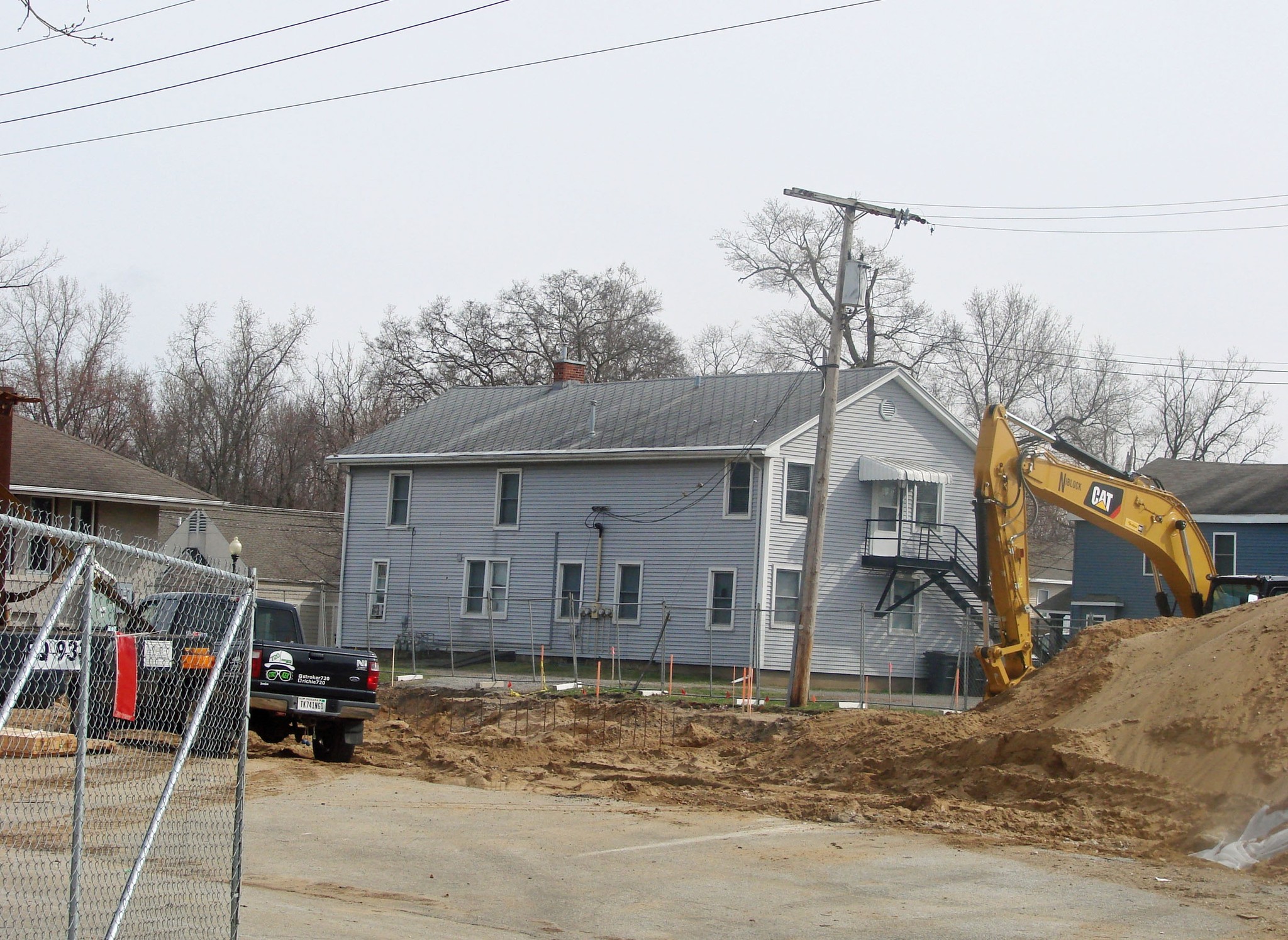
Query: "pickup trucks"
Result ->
[[68, 592, 383, 763]]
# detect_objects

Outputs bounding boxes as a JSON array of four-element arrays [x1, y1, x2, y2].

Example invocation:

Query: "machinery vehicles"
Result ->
[[972, 404, 1288, 702]]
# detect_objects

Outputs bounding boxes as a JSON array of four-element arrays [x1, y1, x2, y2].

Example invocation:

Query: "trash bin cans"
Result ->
[[962, 657, 985, 696], [922, 651, 958, 695]]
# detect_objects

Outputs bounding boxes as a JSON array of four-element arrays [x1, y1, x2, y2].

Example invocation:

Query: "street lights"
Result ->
[[228, 535, 242, 575]]
